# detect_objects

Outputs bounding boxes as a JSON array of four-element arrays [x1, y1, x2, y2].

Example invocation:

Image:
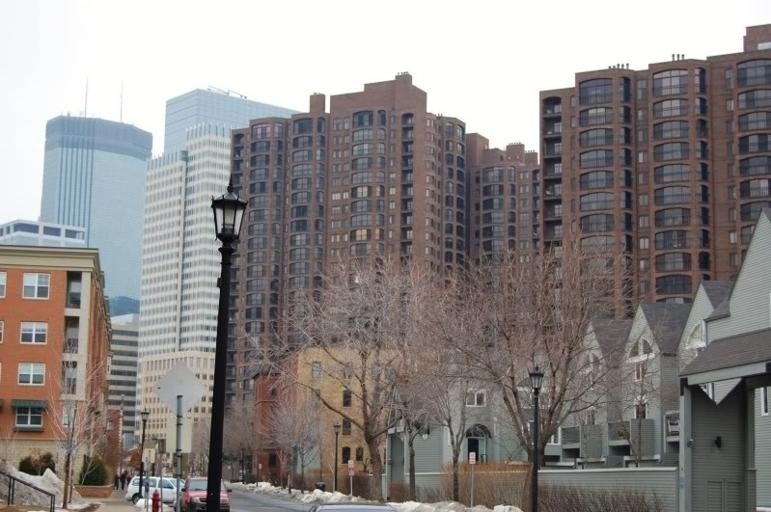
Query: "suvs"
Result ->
[[125, 476, 184, 506], [179, 477, 233, 511]]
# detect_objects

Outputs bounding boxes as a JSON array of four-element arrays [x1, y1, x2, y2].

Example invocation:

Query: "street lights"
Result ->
[[527, 364, 544, 511], [138, 408, 150, 477], [332, 421, 341, 492], [202, 192, 249, 511]]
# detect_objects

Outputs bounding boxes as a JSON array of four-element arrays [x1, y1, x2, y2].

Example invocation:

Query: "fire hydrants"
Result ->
[[149, 490, 160, 512]]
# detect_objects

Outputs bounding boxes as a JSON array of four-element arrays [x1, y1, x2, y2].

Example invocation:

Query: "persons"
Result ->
[[114, 469, 134, 491]]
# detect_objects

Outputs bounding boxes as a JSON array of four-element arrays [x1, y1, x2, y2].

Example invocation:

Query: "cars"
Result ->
[[308, 503, 395, 512]]
